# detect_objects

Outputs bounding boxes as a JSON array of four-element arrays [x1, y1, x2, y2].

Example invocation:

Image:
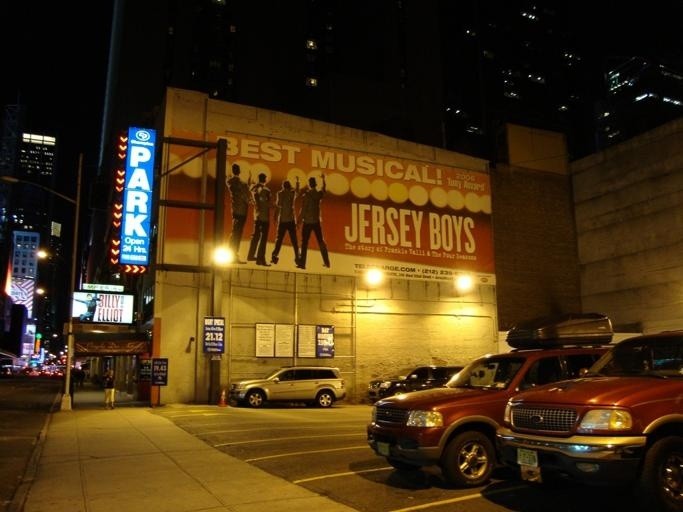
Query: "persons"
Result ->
[[103, 369, 115, 408], [296, 174, 330, 269], [74, 294, 100, 320], [269, 175, 299, 264], [246, 169, 271, 266], [224, 164, 253, 264]]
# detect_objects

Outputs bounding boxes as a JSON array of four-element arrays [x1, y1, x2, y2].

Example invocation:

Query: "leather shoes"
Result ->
[[231, 257, 330, 269]]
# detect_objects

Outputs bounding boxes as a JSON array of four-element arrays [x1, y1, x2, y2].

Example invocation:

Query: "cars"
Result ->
[[1, 365, 63, 378]]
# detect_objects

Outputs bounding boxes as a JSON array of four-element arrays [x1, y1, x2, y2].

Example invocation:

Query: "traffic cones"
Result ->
[[217, 389, 228, 407]]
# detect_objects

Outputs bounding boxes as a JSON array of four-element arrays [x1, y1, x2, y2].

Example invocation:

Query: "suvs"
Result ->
[[228, 366, 348, 407]]
